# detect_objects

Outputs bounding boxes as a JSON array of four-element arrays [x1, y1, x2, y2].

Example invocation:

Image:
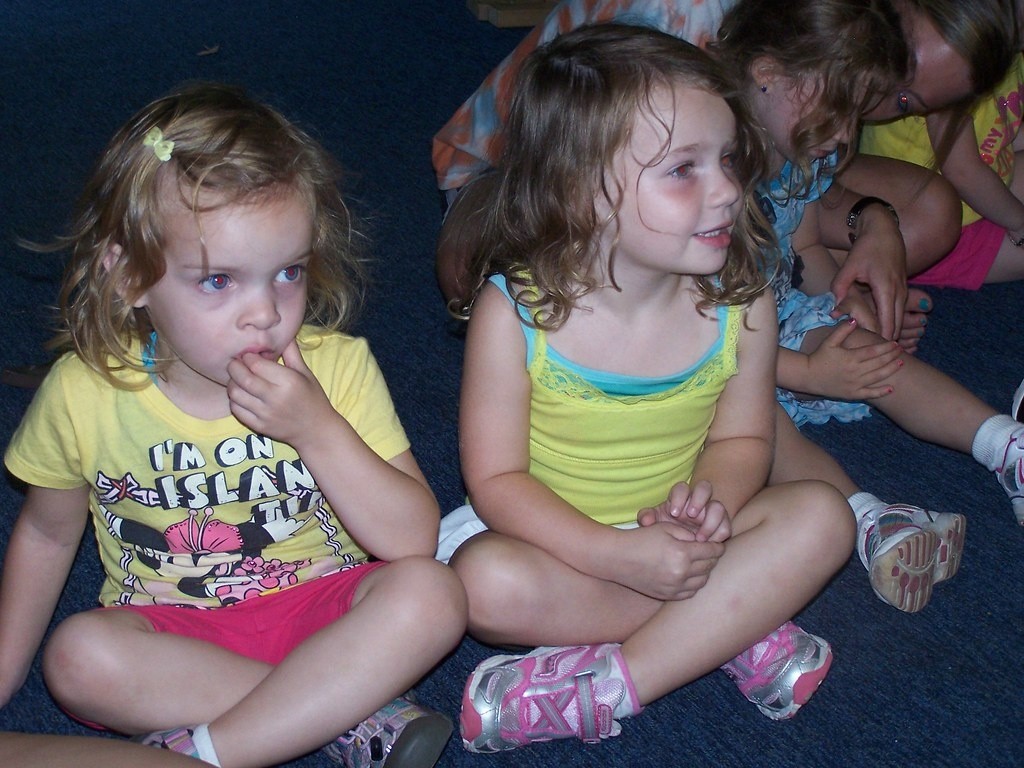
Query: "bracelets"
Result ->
[[1008, 235, 1024, 247], [847, 197, 900, 244]]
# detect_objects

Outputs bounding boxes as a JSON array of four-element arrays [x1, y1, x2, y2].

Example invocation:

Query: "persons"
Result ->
[[857, 48, 1024, 287], [436, 19, 856, 754], [702, 0, 1024, 615], [0, 731, 224, 768], [432, 1, 1024, 356], [0, 84, 472, 767]]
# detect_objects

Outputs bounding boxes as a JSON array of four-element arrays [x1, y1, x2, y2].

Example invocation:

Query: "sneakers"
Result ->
[[995, 429, 1024, 524], [720, 620, 833, 721], [459, 644, 624, 754], [855, 504, 968, 613], [324, 697, 455, 768], [138, 727, 204, 760]]
